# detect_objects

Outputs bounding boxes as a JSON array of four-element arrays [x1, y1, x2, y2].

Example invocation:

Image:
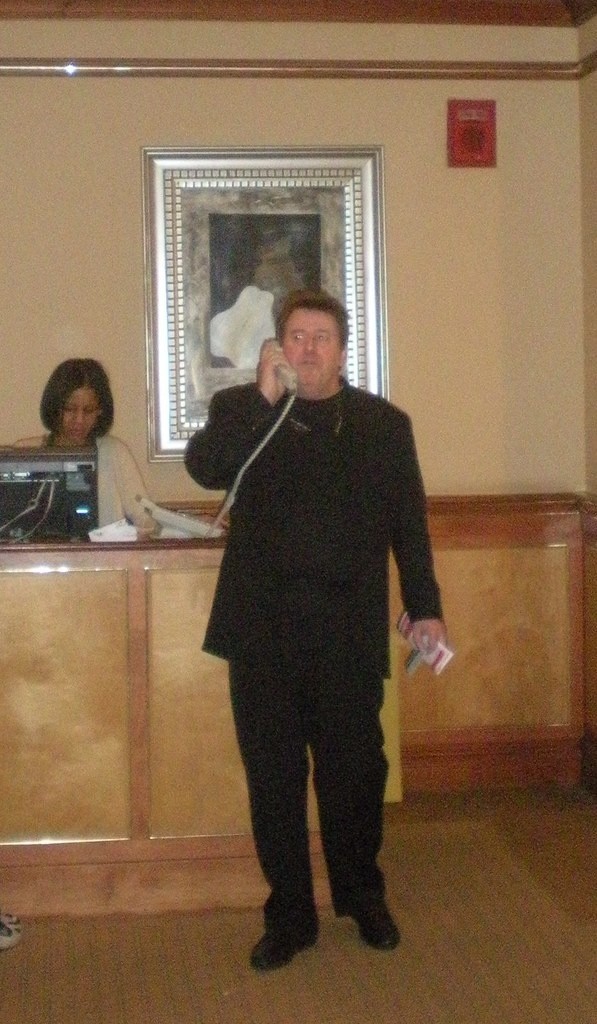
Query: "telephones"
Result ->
[[264, 338, 296, 390]]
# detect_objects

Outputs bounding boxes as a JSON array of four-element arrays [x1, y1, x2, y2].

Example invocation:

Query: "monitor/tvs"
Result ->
[[0, 445, 98, 542]]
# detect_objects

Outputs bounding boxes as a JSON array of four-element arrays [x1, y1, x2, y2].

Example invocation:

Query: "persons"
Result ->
[[13, 357, 159, 529], [182, 293, 447, 970]]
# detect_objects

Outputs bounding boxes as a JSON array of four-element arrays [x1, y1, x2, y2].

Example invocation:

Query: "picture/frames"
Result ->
[[139, 142, 389, 466]]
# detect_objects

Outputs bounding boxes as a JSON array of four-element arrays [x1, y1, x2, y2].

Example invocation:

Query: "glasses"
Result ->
[[282, 329, 345, 349]]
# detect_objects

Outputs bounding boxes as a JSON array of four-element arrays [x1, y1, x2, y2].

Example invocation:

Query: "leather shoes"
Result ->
[[351, 901, 402, 949], [249, 923, 321, 971]]
